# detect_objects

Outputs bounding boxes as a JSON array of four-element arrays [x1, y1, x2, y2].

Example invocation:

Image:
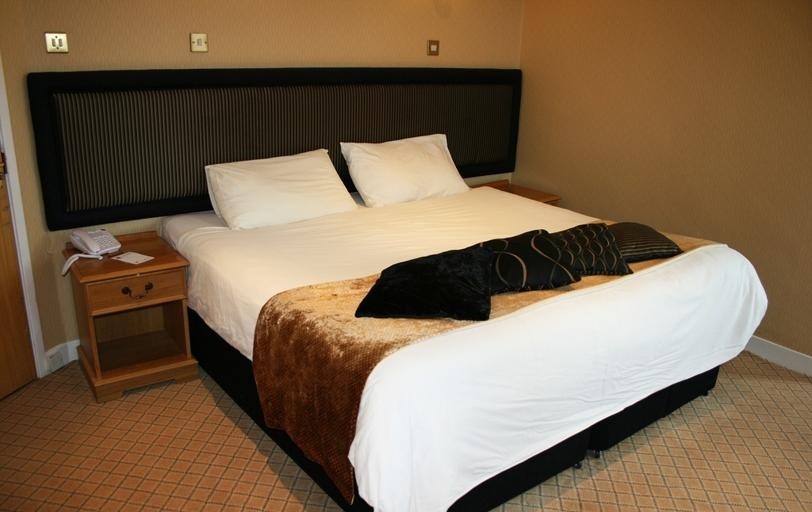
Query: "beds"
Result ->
[[26, 67, 769, 512]]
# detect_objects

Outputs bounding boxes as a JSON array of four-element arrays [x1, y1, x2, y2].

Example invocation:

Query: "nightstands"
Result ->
[[470, 179, 561, 207], [62, 230, 201, 403]]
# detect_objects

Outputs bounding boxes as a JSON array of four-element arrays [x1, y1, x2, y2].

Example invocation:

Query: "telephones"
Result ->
[[69, 227, 121, 256]]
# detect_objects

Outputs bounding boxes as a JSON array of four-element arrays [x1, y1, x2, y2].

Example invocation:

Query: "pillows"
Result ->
[[354, 243, 493, 321], [471, 227, 583, 295], [340, 134, 470, 209], [205, 149, 357, 230], [608, 221, 684, 264], [550, 219, 632, 278]]
